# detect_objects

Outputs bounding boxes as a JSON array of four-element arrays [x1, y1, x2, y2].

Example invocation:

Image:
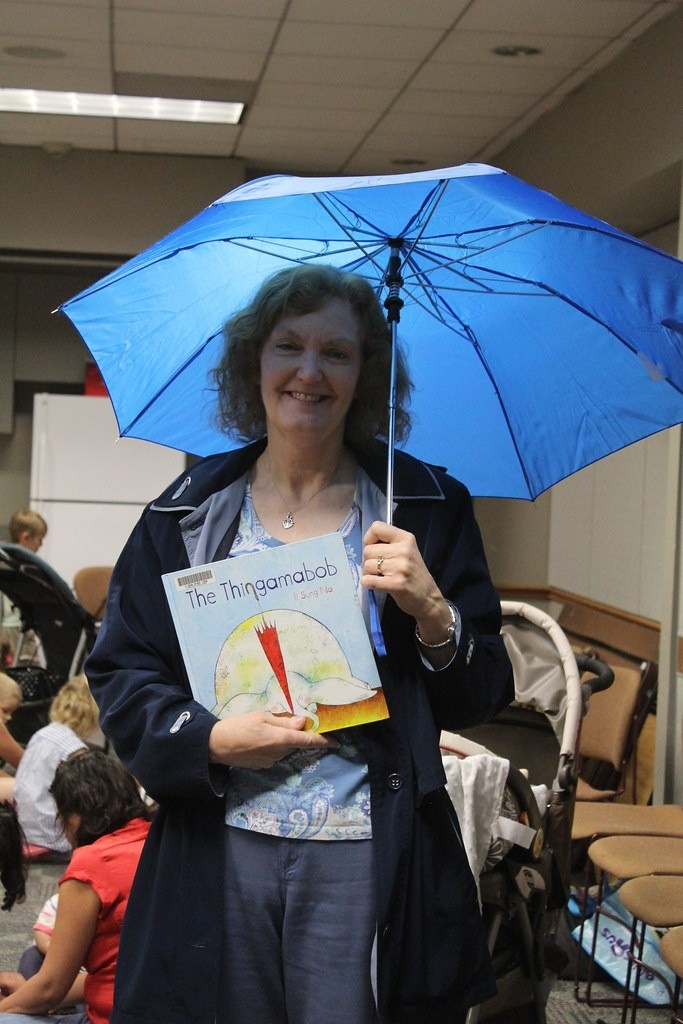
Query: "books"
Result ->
[[159, 531, 390, 735]]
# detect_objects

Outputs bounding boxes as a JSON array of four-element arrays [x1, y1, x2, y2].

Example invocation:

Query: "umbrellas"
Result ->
[[49, 162, 683, 524]]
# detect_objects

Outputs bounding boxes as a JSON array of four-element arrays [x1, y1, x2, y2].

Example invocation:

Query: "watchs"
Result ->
[[415, 606, 455, 648]]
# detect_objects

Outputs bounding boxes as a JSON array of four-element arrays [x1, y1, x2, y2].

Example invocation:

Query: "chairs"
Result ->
[[571, 646, 683, 1024]]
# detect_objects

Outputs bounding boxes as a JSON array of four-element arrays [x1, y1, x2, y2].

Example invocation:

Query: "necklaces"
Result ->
[[265, 443, 341, 529]]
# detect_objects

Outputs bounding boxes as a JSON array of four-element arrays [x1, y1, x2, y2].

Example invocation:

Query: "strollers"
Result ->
[[0, 544, 108, 776], [436, 603, 615, 1023]]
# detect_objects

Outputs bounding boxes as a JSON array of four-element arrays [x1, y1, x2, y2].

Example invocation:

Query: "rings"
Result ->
[[376, 554, 384, 575]]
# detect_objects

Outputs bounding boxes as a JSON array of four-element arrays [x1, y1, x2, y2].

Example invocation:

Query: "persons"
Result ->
[[80, 264, 517, 1024], [0, 510, 48, 669], [0, 672, 27, 916], [14, 672, 100, 864], [14, 892, 91, 1017], [0, 750, 154, 1024]]
